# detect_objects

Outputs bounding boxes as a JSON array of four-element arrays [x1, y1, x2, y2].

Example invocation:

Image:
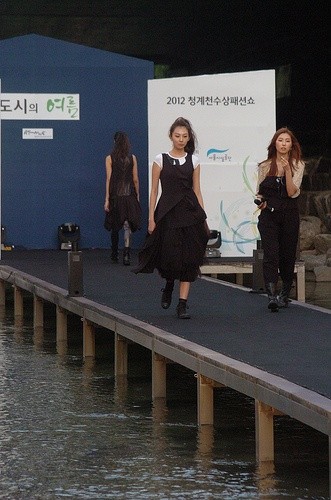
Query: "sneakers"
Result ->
[[161, 288, 172, 309], [176, 303, 191, 319]]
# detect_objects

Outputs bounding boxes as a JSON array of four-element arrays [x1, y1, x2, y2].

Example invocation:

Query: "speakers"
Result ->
[[252, 249, 266, 292], [68, 251, 83, 297]]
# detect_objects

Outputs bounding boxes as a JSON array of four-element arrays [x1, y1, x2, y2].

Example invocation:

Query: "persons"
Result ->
[[255, 128, 306, 312], [131, 116, 210, 319], [103, 131, 142, 265]]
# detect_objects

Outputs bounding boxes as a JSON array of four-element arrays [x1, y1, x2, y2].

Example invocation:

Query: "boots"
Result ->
[[277, 283, 291, 308], [265, 282, 279, 312]]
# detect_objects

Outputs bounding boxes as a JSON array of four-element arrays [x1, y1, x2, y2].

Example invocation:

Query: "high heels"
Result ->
[[111, 247, 119, 263], [123, 247, 131, 265]]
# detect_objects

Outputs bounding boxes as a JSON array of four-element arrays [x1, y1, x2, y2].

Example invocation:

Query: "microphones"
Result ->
[[254, 199, 274, 212]]
